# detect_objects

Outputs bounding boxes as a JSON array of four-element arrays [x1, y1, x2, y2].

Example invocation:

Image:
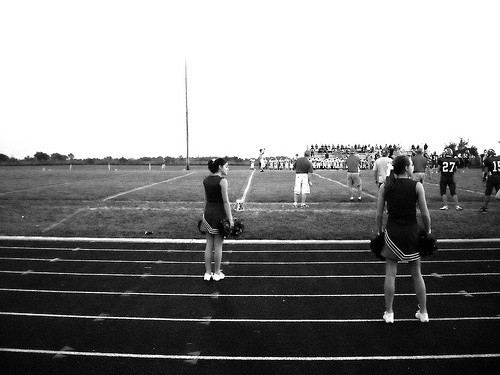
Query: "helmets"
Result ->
[[488, 149, 496, 155], [445, 148, 453, 156]]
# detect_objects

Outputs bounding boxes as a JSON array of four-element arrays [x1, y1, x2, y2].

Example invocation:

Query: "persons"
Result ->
[[346, 149, 362, 201], [438, 148, 462, 210], [202, 158, 234, 281], [248, 154, 300, 171], [311, 144, 349, 170], [376, 155, 437, 323], [362, 144, 381, 170], [293, 150, 313, 208], [479, 149, 500, 214], [430, 149, 470, 174], [411, 149, 428, 184], [385, 143, 429, 155], [259, 148, 266, 172], [373, 148, 393, 213]]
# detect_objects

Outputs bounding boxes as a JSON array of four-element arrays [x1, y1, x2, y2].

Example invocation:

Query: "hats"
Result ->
[[347, 148, 354, 153], [382, 147, 388, 155], [415, 147, 423, 154]]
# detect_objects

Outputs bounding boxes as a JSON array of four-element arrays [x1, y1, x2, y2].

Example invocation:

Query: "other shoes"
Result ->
[[358, 196, 361, 200], [213, 270, 226, 281], [292, 202, 297, 208], [481, 206, 488, 214], [349, 196, 354, 200], [301, 204, 309, 209], [204, 271, 213, 281]]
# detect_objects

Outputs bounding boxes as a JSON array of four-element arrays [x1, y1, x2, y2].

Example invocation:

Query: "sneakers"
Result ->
[[455, 205, 462, 210], [383, 311, 394, 323], [415, 303, 429, 323], [439, 205, 448, 210]]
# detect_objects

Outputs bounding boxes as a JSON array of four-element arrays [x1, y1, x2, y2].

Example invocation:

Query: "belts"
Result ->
[[347, 170, 358, 173]]
